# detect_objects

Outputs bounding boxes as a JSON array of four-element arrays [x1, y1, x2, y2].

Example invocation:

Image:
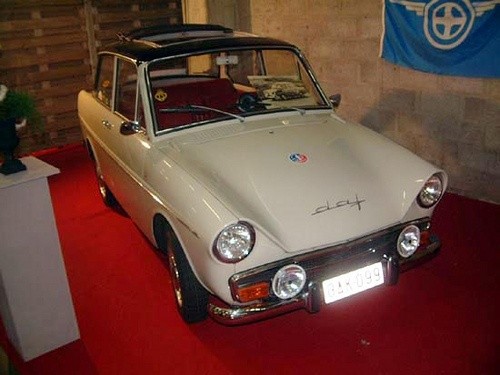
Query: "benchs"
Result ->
[[119, 78, 242, 131]]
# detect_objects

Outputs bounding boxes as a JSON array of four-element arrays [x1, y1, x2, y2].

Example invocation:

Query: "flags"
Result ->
[[380, 0, 500, 79]]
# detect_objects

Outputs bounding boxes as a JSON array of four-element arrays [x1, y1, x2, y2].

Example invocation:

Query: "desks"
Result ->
[[0, 155, 80, 361]]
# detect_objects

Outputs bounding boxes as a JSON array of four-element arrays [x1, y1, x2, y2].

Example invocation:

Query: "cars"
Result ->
[[77, 23, 450, 326]]
